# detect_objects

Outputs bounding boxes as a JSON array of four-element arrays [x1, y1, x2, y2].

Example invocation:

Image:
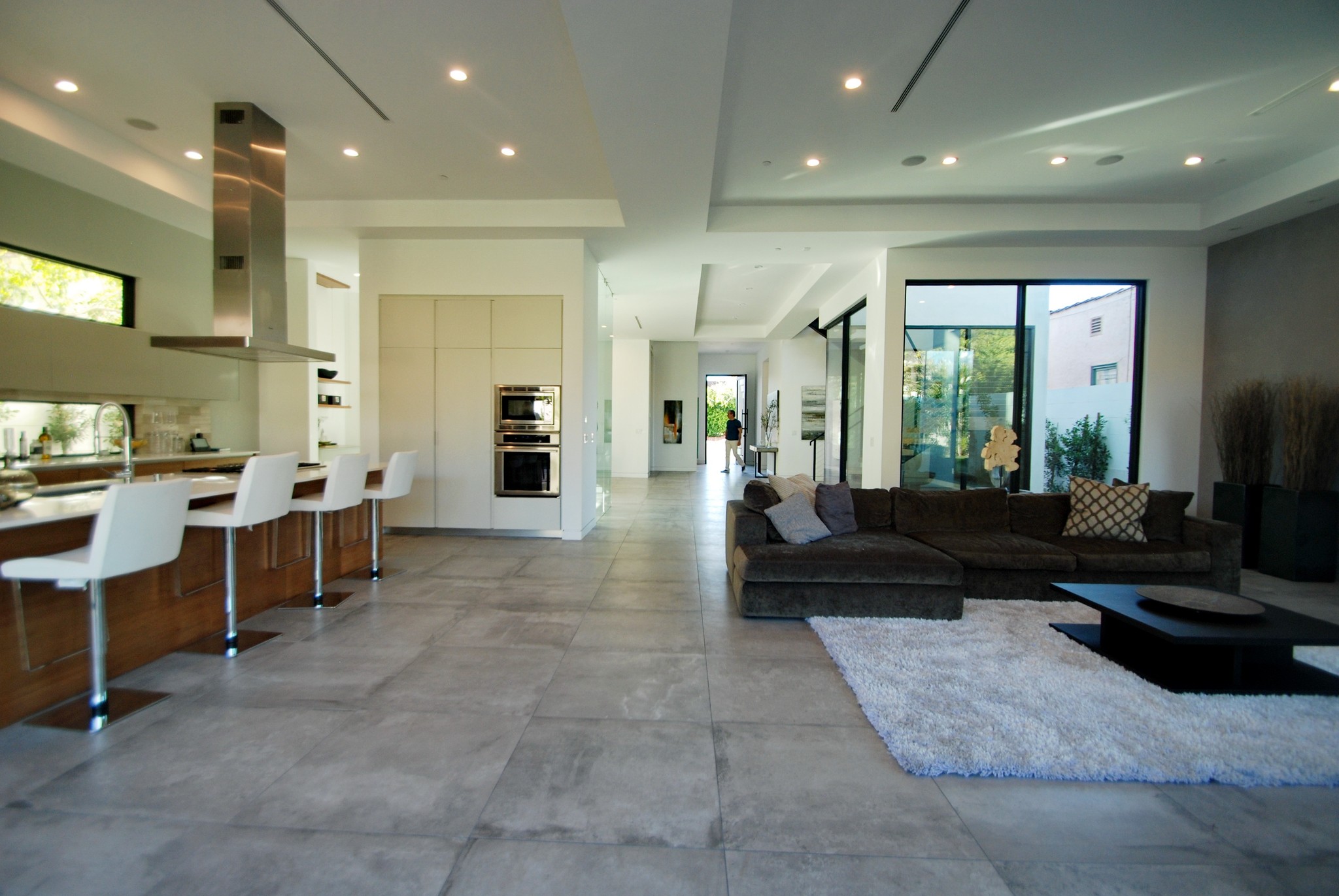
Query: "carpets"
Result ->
[[806, 598, 1339, 786]]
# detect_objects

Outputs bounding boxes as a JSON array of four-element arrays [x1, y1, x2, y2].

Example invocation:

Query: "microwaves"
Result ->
[[494, 385, 561, 432]]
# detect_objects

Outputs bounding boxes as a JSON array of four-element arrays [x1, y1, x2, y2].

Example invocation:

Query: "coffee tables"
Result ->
[[1049, 583, 1339, 694]]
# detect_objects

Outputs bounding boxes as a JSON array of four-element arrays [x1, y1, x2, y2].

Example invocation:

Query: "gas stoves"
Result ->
[[181, 462, 321, 473]]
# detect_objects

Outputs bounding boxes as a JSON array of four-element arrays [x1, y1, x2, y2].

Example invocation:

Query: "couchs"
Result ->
[[725, 480, 1245, 619]]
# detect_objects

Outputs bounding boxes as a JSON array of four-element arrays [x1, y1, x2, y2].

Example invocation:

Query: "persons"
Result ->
[[721, 410, 746, 473]]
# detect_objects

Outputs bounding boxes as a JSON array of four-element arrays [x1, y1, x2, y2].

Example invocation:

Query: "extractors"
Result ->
[[150, 101, 335, 363]]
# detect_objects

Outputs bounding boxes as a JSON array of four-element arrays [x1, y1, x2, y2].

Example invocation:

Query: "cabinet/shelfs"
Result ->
[[380, 295, 564, 537], [319, 377, 352, 448]]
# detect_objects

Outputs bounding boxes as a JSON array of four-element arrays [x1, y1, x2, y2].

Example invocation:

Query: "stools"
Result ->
[[278, 453, 369, 609], [342, 450, 421, 581], [0, 478, 189, 734], [175, 452, 301, 657]]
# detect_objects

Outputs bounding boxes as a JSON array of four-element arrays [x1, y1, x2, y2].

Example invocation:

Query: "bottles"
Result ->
[[19, 432, 29, 459], [29, 439, 43, 461], [147, 432, 185, 454], [39, 427, 52, 457]]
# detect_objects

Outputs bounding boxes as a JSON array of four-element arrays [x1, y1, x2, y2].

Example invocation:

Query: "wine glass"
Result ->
[[164, 411, 178, 435], [151, 411, 164, 434]]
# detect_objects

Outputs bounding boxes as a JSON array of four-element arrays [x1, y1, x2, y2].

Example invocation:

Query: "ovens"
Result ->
[[493, 432, 560, 498]]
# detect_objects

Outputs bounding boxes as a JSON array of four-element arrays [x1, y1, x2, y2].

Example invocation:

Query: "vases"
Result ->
[[764, 432, 772, 447]]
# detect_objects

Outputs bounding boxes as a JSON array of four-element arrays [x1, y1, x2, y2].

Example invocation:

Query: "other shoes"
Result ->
[[721, 469, 729, 472], [742, 465, 746, 472]]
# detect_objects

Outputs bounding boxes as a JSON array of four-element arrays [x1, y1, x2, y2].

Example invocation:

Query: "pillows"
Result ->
[[1061, 475, 1150, 543], [765, 472, 859, 544]]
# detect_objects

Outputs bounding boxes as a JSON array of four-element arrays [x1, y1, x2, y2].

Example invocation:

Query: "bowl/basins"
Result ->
[[112, 437, 150, 452], [318, 368, 338, 379]]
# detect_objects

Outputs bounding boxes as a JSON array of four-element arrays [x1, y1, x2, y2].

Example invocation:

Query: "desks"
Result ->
[[750, 445, 778, 478]]
